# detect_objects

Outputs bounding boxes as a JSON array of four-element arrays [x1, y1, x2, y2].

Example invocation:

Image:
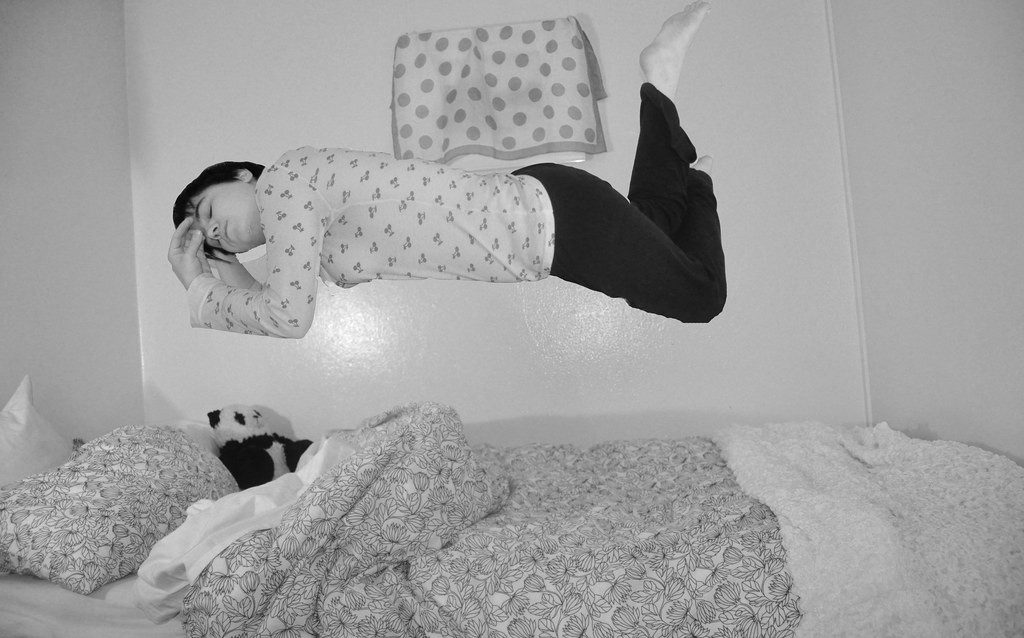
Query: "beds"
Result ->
[[0, 421, 1024, 638]]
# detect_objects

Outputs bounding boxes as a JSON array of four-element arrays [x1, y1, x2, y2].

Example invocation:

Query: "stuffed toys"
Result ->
[[207, 405, 314, 489]]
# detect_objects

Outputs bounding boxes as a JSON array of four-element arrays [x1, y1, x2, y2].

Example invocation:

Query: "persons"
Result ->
[[168, 0, 729, 339]]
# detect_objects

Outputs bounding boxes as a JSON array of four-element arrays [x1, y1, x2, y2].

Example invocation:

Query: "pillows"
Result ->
[[0, 423, 240, 597], [0, 374, 72, 486]]
